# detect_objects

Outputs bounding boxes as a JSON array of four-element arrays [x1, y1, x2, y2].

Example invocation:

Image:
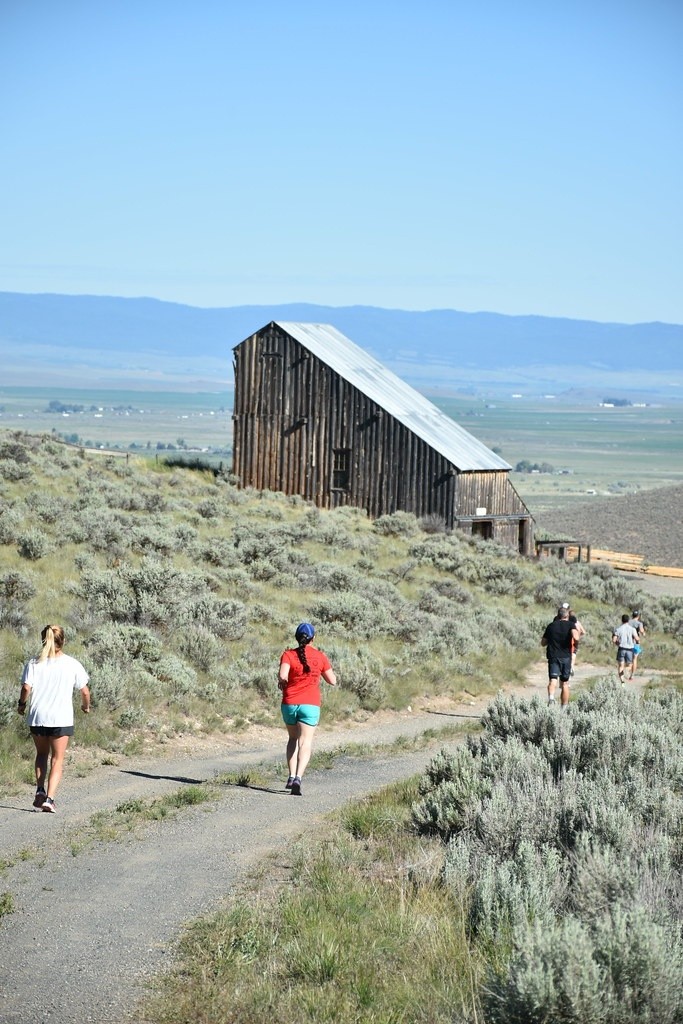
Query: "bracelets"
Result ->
[[18, 699, 27, 706]]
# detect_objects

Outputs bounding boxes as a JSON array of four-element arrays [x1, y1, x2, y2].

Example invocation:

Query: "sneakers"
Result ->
[[32, 787, 47, 808], [286, 777, 294, 789], [291, 777, 302, 796], [41, 797, 56, 813]]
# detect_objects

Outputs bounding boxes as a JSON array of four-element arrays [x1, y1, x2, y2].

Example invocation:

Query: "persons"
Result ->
[[541, 608, 579, 709], [628, 611, 645, 679], [553, 603, 586, 688], [277, 623, 336, 796], [17, 626, 90, 813], [612, 614, 640, 687]]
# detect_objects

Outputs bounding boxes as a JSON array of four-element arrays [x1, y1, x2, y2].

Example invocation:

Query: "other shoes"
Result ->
[[548, 699, 555, 707]]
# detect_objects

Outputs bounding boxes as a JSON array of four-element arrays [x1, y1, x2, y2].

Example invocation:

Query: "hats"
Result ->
[[295, 622, 314, 641], [562, 603, 570, 609], [633, 610, 639, 615]]
